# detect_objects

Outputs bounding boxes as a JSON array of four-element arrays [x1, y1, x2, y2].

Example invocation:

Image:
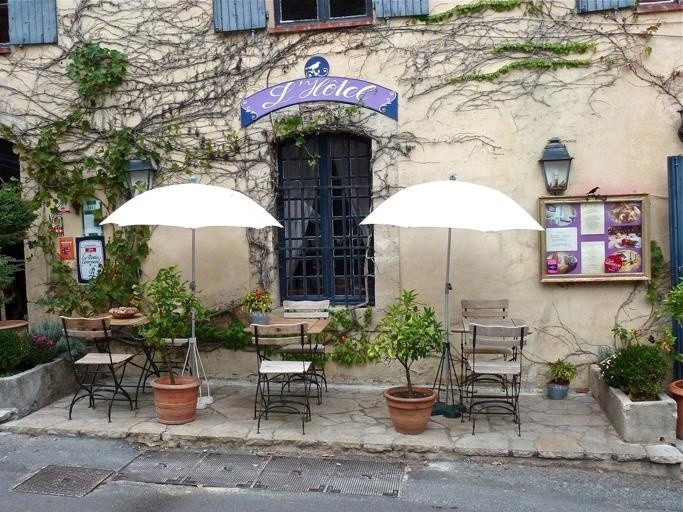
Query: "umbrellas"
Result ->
[[359, 177, 544, 405], [99, 178, 284, 378]]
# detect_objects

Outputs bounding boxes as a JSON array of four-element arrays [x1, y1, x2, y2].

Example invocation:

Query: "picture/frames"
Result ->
[[536, 193, 653, 283]]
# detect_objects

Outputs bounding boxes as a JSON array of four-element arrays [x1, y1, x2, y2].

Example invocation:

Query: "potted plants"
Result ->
[[659, 276, 683, 440], [131, 261, 204, 426], [241, 288, 273, 326], [367, 277, 446, 435], [602, 341, 677, 445], [542, 357, 577, 400]]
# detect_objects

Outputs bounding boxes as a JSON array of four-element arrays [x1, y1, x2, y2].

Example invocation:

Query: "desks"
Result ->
[[449, 317, 530, 420], [86, 310, 160, 409], [242, 314, 328, 407]]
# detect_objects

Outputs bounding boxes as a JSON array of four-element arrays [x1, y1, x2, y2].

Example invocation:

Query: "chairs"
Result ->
[[278, 299, 330, 405], [58, 315, 133, 422], [459, 297, 511, 404], [141, 294, 198, 393], [466, 320, 528, 438], [248, 322, 312, 435]]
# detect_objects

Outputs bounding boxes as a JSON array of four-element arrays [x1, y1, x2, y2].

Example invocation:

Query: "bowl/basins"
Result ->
[[108, 308, 138, 319]]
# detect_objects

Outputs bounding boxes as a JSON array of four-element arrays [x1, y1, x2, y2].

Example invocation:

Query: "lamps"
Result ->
[[537, 136, 574, 191], [119, 152, 159, 198]]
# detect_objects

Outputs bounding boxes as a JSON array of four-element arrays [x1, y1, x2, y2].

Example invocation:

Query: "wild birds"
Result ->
[[586, 186, 600, 195]]
